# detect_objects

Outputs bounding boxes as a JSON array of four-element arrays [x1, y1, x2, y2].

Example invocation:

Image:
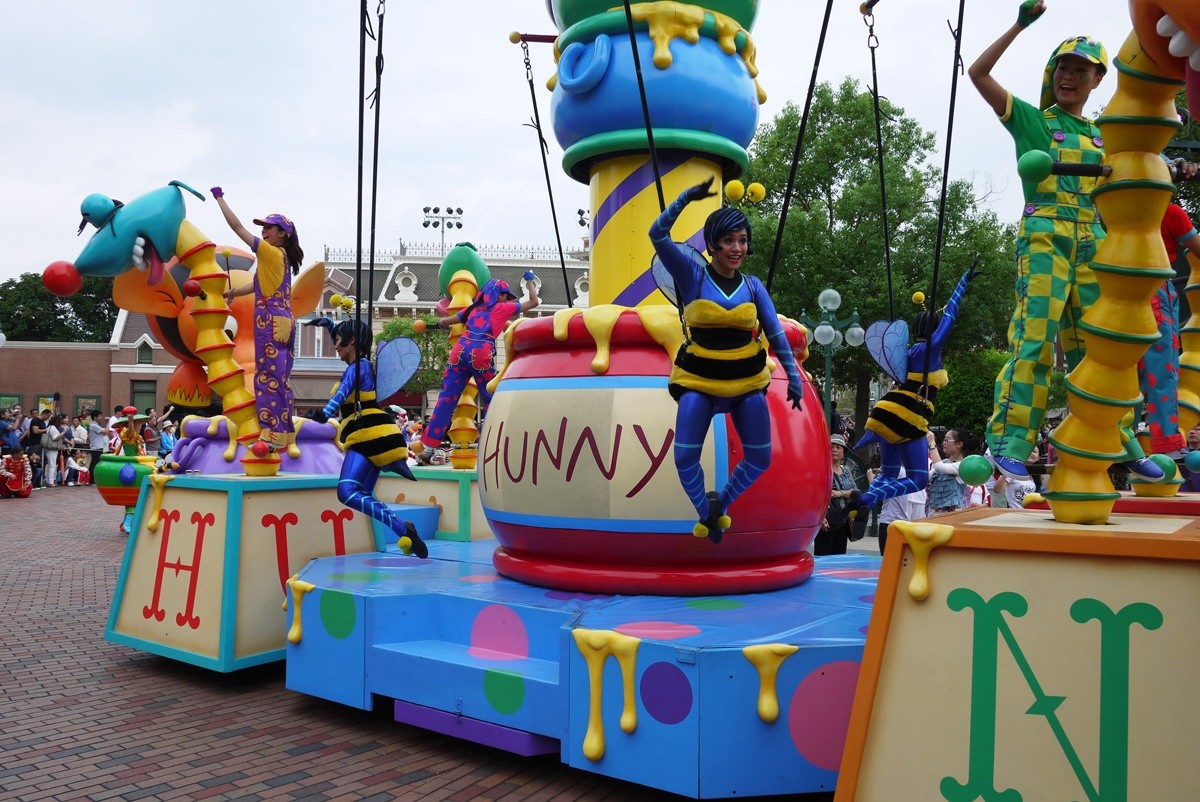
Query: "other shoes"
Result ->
[[38, 485, 46, 489], [68, 482, 74, 487], [704, 489, 724, 543], [401, 518, 427, 558], [853, 506, 870, 538], [46, 482, 57, 487], [841, 486, 863, 518], [31, 487, 41, 491]]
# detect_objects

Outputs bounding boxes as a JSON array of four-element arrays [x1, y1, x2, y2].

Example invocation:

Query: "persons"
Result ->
[[814, 434, 862, 556], [839, 267, 974, 519], [211, 186, 304, 449], [303, 318, 428, 559], [647, 173, 804, 545], [381, 405, 431, 451], [967, 0, 1165, 483], [0, 404, 205, 498], [867, 421, 1200, 557], [418, 269, 539, 465]]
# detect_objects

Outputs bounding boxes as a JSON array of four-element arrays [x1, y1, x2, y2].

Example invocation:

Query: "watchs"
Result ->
[[928, 443, 937, 450]]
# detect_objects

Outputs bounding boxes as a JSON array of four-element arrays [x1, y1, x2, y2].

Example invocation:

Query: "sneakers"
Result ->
[[1125, 457, 1166, 482], [993, 455, 1030, 481]]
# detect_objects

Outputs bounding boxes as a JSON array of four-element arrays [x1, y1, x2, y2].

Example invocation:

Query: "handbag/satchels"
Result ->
[[40, 425, 64, 450]]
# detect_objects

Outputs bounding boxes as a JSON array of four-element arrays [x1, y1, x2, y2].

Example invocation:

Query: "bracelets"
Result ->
[[840, 490, 845, 497]]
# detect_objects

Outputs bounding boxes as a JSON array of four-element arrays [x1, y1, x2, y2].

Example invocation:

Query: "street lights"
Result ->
[[421, 204, 466, 260], [797, 279, 878, 439]]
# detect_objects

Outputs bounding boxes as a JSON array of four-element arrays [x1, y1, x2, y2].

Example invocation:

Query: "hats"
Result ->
[[829, 434, 846, 447], [162, 420, 174, 429], [111, 406, 154, 428], [1, 408, 8, 413], [253, 212, 293, 232], [1051, 34, 1108, 74]]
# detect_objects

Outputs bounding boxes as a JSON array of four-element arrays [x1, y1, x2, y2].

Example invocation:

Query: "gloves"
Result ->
[[211, 186, 224, 199]]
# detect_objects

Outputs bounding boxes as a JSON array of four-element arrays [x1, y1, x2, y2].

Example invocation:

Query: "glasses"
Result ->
[[943, 438, 957, 444]]
